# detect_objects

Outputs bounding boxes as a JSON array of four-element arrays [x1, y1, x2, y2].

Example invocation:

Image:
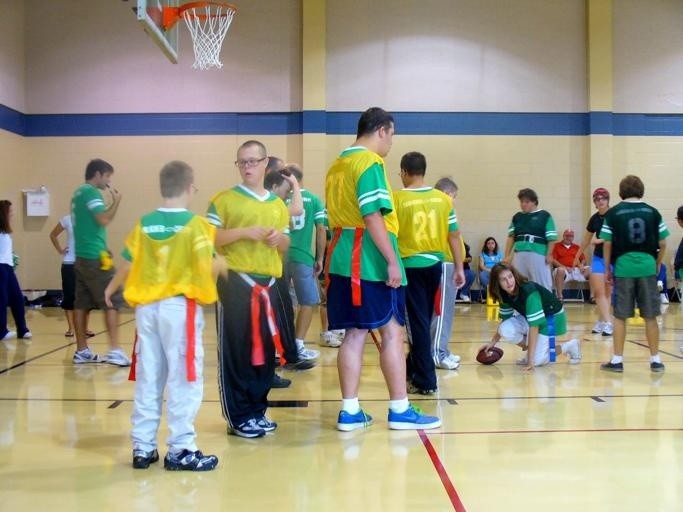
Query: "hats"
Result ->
[[564, 229, 575, 236]]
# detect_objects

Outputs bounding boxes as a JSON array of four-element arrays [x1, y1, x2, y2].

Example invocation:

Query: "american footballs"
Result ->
[[476, 346, 504, 365]]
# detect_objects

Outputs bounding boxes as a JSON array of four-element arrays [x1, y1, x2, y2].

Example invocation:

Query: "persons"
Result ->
[[674, 206, 683, 291], [0, 199, 33, 339], [49, 213, 95, 337], [551, 228, 596, 304], [485, 261, 581, 370], [69, 159, 133, 367], [263, 157, 349, 389], [598, 177, 671, 371], [656, 263, 669, 304], [573, 188, 614, 336], [430, 178, 466, 370], [208, 140, 292, 439], [478, 237, 505, 303], [391, 152, 467, 394], [325, 106, 443, 430], [501, 188, 558, 294], [103, 161, 228, 471], [458, 241, 475, 304]]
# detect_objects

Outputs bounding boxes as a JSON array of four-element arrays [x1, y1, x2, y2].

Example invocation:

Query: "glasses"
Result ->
[[235, 157, 266, 168]]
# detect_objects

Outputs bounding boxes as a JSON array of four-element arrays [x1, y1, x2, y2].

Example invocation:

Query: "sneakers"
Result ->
[[226, 329, 461, 438], [569, 320, 623, 373], [3, 330, 131, 366], [460, 293, 469, 301], [651, 362, 664, 371], [164, 449, 217, 470], [133, 449, 159, 468]]
